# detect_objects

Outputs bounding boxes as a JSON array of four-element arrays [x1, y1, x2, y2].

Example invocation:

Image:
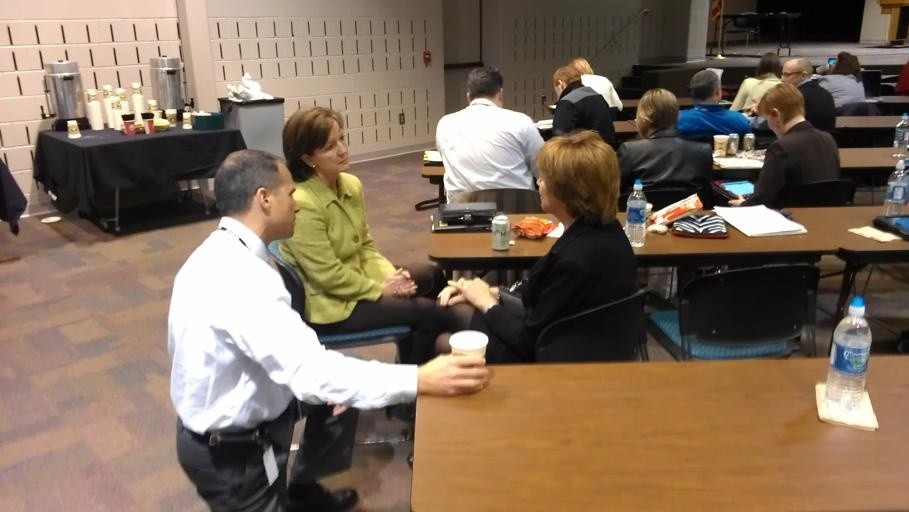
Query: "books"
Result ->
[[652, 194, 727, 237]]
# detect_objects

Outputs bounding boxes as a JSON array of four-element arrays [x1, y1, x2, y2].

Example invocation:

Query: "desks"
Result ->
[[428, 205, 909, 287], [410, 355, 909, 512], [32, 120, 248, 235], [710, 11, 803, 57], [420, 83, 909, 207]]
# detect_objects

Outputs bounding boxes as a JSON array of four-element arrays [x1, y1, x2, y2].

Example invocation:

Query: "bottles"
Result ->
[[626, 179, 647, 248], [884, 156, 909, 216], [824, 295, 873, 406], [890, 112, 909, 158]]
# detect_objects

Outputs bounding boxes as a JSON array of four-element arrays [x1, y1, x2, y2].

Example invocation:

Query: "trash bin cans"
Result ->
[[217, 97, 284, 158]]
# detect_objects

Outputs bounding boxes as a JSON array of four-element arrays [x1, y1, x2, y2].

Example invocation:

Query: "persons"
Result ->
[[551, 57, 623, 150], [434, 68, 545, 192], [727, 82, 842, 208], [278, 105, 448, 422], [677, 53, 865, 132], [166, 148, 489, 512], [617, 89, 716, 197], [402, 128, 644, 471]]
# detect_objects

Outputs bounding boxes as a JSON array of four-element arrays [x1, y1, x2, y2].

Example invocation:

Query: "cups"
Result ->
[[645, 201, 653, 228], [713, 134, 729, 158], [447, 328, 490, 362], [85, 82, 193, 135], [66, 119, 82, 139]]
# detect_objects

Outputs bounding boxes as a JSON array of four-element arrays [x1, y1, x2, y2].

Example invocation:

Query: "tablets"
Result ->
[[828, 57, 836, 65], [711, 178, 755, 203], [872, 214, 909, 243]]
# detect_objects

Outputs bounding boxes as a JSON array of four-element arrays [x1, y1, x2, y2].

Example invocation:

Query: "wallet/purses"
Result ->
[[440, 202, 498, 225]]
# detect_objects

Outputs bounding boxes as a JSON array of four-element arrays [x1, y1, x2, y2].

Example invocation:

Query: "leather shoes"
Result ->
[[286, 483, 357, 512]]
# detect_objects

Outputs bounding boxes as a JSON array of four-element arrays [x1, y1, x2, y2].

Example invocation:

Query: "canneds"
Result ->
[[743, 133, 755, 150], [492, 215, 510, 251], [727, 133, 740, 154]]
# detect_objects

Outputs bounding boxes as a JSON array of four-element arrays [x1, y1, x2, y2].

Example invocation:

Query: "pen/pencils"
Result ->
[[713, 161, 721, 166], [447, 218, 489, 225]]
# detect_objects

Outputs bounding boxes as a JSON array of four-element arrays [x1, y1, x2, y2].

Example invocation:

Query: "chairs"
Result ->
[[534, 286, 653, 362], [266, 236, 411, 363], [860, 69, 882, 96], [799, 247, 909, 356], [648, 262, 820, 359], [723, 11, 761, 49]]
